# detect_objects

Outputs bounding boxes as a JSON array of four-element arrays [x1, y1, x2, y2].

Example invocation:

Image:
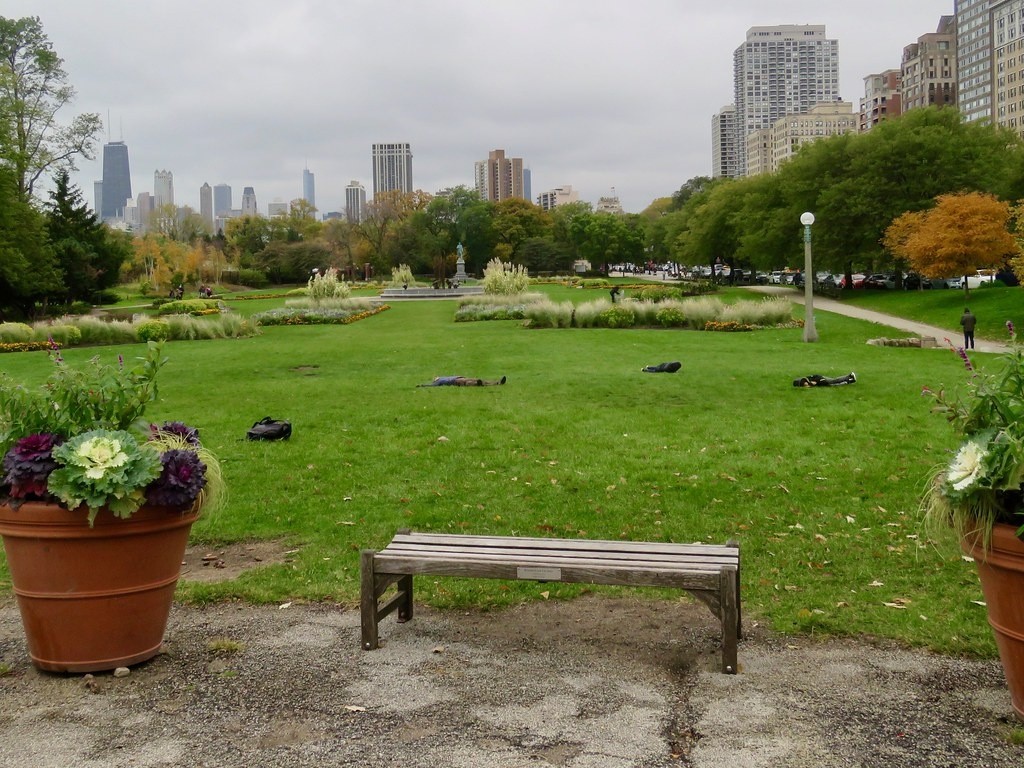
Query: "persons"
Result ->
[[793, 372, 856, 387], [960, 308, 977, 351], [198, 284, 206, 296], [176, 286, 184, 300], [780, 273, 787, 285], [169, 289, 175, 300], [417, 374, 506, 386], [456, 241, 463, 258], [402, 279, 407, 290], [640, 361, 681, 373], [431, 275, 459, 289], [610, 287, 621, 303], [206, 286, 212, 296]]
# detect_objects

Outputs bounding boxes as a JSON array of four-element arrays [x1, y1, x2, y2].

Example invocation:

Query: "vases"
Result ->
[[960, 519, 1024, 724], [0, 501, 200, 673]]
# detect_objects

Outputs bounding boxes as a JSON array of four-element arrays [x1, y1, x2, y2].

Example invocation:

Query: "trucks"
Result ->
[[613, 263, 639, 272]]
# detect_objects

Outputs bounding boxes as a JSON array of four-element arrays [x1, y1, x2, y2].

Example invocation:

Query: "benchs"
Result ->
[[361, 530, 742, 674]]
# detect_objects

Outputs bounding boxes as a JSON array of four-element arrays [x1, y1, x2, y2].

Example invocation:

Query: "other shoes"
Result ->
[[641, 368, 644, 372], [971, 348, 974, 350], [477, 379, 484, 387], [645, 365, 648, 370], [612, 301, 614, 302], [498, 376, 506, 385], [845, 372, 856, 384], [964, 348, 968, 350]]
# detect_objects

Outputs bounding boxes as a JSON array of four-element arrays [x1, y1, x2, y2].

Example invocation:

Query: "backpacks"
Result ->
[[247, 416, 292, 441]]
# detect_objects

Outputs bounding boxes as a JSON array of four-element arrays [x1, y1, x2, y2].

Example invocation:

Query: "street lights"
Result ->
[[800, 212, 817, 342]]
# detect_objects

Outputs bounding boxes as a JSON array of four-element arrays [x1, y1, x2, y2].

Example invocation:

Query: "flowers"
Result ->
[[0, 334, 229, 526], [915, 320, 1024, 566]]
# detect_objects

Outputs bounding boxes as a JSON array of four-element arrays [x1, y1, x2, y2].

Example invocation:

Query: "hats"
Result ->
[[796, 269, 800, 273], [901, 273, 908, 279], [909, 269, 915, 273], [965, 308, 969, 312]]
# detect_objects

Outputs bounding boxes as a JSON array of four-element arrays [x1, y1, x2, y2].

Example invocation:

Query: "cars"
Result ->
[[960, 270, 995, 288], [930, 276, 961, 289], [736, 269, 751, 281], [793, 273, 805, 285], [904, 273, 931, 290], [780, 272, 793, 285], [825, 274, 844, 288], [883, 273, 908, 290], [748, 271, 767, 283], [692, 264, 730, 276], [769, 271, 783, 283], [841, 273, 866, 289], [865, 274, 887, 288]]
